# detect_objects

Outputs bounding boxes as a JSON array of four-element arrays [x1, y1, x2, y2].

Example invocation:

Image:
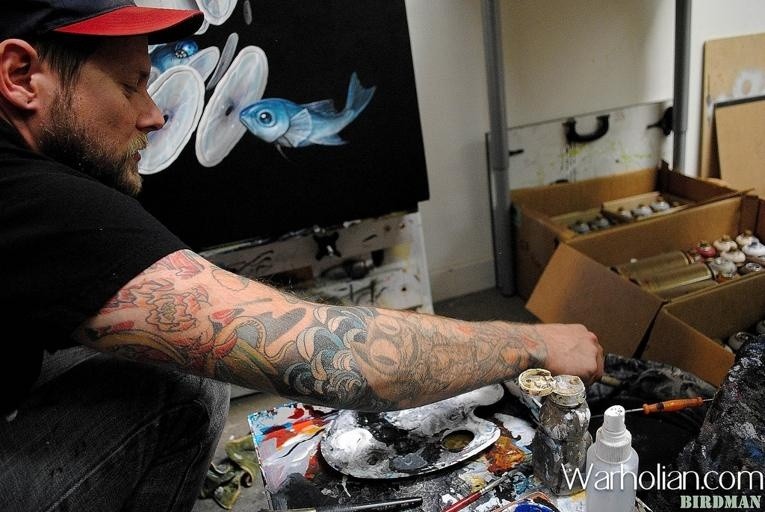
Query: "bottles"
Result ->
[[606, 228, 765, 304], [568, 193, 672, 236], [584, 404, 638, 511], [518, 366, 593, 496]]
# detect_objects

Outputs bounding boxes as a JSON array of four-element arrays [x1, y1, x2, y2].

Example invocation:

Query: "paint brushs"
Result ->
[[259, 496, 423, 512], [594, 376, 631, 389], [441, 468, 519, 512]]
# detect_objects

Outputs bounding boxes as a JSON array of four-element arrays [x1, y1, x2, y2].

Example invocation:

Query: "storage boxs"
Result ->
[[509, 166, 765, 389]]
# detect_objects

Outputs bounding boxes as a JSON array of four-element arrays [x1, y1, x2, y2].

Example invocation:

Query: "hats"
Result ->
[[0, 0, 203, 45]]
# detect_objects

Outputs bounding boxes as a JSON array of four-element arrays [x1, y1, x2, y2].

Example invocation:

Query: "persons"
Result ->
[[0, 1, 610, 512]]
